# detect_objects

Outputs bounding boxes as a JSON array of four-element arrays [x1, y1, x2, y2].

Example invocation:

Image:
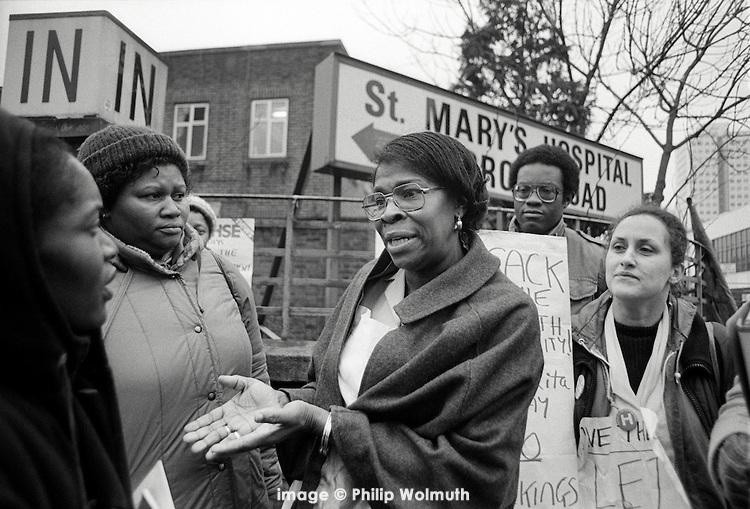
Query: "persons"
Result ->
[[181, 130, 544, 509], [74, 123, 283, 509], [186, 195, 216, 247], [573, 203, 740, 509], [1, 107, 132, 509], [509, 140, 609, 319]]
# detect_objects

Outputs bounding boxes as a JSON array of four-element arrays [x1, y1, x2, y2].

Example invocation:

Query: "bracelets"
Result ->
[[319, 409, 337, 457]]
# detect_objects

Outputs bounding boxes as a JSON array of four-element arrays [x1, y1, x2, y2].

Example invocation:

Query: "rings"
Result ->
[[225, 425, 231, 436], [234, 431, 241, 440]]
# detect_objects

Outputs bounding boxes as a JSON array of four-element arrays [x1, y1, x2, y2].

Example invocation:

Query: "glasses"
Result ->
[[362, 183, 445, 221], [513, 184, 566, 202]]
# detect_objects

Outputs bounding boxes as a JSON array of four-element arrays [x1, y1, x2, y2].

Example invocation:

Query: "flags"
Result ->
[[686, 196, 738, 322]]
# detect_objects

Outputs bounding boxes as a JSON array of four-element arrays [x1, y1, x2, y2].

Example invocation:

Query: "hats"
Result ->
[[187, 198, 217, 239], [77, 124, 189, 181]]
